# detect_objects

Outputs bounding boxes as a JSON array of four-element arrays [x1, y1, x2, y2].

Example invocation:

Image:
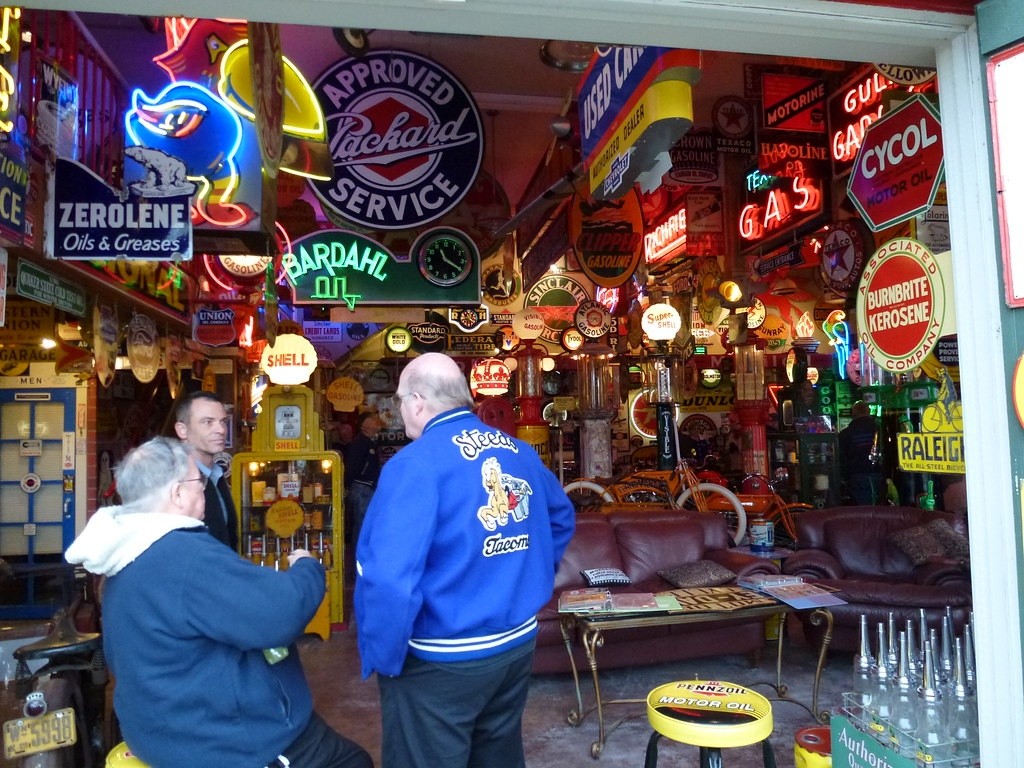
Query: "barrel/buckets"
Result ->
[[749, 522, 774, 553], [793, 726, 832, 768]]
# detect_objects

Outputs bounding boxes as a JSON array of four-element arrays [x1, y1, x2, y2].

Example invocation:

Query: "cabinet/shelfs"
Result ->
[[230, 450, 347, 625], [767, 432, 842, 510]]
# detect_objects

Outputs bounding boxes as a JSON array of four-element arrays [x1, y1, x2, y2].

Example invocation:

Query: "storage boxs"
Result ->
[[828, 713, 922, 768]]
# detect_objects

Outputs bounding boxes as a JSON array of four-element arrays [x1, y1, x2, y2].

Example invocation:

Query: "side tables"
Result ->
[[727, 546, 795, 641]]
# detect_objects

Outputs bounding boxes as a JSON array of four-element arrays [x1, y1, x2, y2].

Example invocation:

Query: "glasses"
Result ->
[[390, 392, 426, 409], [179, 474, 209, 487]]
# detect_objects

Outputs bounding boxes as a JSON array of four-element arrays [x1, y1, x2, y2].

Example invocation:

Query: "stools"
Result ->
[[644, 680, 778, 768]]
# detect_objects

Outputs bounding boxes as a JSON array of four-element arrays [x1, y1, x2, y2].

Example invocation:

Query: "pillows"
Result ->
[[656, 560, 738, 590], [580, 567, 633, 589], [888, 517, 974, 567]]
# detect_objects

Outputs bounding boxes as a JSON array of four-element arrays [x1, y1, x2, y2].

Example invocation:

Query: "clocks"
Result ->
[[418, 234, 473, 289], [541, 400, 570, 432]]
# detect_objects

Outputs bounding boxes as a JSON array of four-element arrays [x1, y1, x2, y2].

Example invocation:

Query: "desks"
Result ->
[[558, 586, 832, 760]]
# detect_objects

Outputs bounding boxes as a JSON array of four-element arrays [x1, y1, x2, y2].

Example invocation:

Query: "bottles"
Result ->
[[851, 605, 980, 768]]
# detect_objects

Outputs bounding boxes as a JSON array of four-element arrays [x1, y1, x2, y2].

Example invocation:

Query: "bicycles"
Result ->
[[2, 561, 105, 768], [562, 457, 814, 551]]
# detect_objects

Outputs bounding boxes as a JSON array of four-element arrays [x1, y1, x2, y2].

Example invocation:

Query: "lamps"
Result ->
[[280, 134, 336, 181], [331, 24, 377, 57], [538, 39, 594, 72]]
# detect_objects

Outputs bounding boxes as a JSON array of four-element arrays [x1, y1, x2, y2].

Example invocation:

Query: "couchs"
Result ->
[[781, 506, 973, 669], [527, 510, 781, 675]]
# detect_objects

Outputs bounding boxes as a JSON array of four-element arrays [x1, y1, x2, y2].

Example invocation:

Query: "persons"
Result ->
[[102, 435, 374, 768], [344, 417, 381, 528], [334, 425, 353, 458], [355, 351, 575, 768], [838, 400, 888, 503], [176, 391, 239, 552]]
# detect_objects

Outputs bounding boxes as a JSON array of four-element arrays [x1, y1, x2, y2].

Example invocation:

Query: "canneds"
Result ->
[[748, 518, 775, 552]]
